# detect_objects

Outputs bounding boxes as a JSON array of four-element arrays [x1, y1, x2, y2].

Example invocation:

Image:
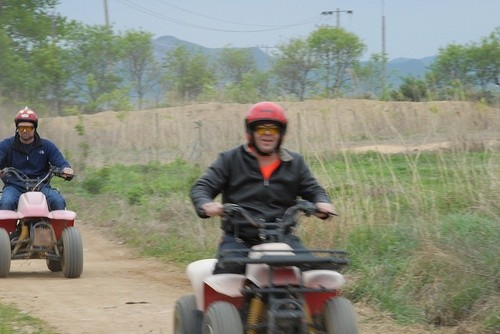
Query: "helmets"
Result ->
[[245, 102, 287, 150], [15, 108, 38, 129]]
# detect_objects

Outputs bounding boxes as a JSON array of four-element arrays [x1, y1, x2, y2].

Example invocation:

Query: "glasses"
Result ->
[[17, 126, 35, 132], [251, 124, 282, 136]]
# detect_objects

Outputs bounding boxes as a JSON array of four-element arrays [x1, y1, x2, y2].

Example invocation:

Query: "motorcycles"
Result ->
[[0, 168, 83, 279], [172, 200, 360, 334]]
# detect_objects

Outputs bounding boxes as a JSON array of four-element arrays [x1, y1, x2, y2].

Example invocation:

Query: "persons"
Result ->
[[0, 106, 73, 211], [188, 102, 341, 275]]
